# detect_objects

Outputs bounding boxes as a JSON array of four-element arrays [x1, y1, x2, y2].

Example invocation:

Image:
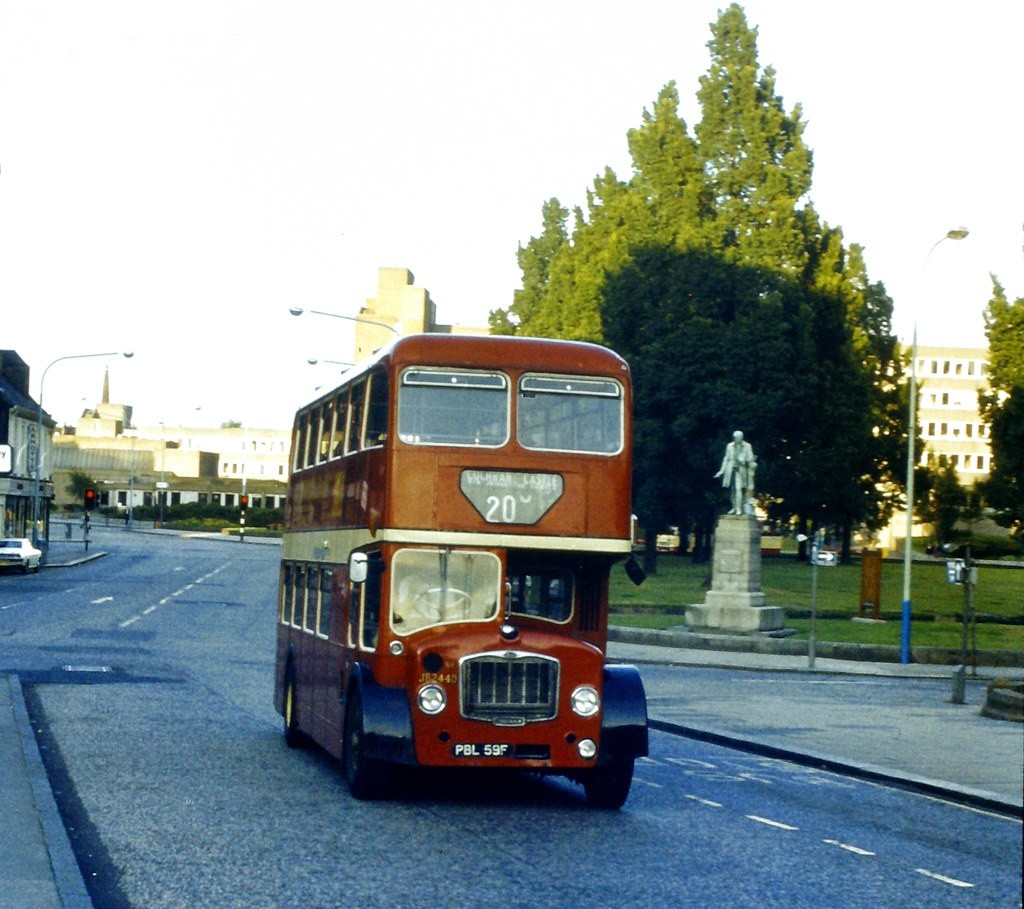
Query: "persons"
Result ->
[[714, 431, 754, 515], [399, 555, 461, 621]]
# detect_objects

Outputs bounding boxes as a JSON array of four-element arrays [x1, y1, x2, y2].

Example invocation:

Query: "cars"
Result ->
[[0, 538, 42, 575]]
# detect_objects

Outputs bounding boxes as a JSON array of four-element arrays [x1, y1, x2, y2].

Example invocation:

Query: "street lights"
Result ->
[[897, 226, 969, 666], [30, 349, 134, 549]]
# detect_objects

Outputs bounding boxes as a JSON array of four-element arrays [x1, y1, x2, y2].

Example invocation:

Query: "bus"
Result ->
[[655, 514, 784, 556], [271, 335, 651, 813]]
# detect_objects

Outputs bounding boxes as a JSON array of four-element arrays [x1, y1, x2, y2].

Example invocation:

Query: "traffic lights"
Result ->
[[240, 496, 248, 510], [85, 489, 94, 509]]
